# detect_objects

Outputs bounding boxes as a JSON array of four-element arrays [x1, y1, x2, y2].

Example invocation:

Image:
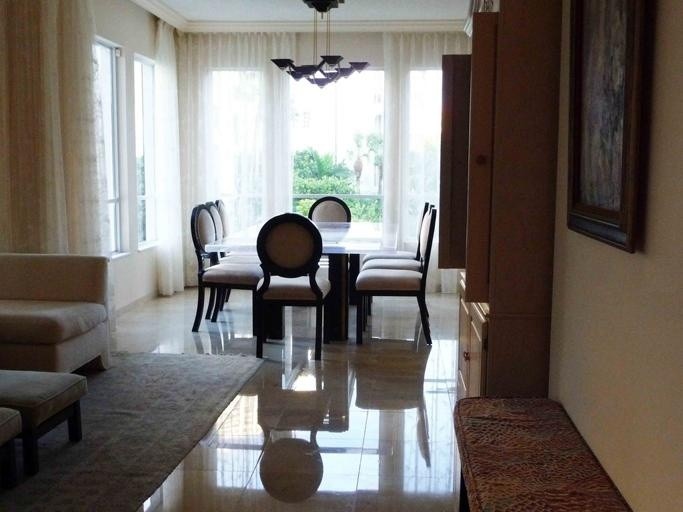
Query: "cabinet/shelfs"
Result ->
[[435, 2, 562, 398]]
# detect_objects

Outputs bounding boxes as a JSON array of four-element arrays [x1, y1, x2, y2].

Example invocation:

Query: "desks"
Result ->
[[205, 220, 383, 345]]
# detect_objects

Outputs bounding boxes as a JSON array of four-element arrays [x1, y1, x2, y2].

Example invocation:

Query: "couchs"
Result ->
[[0, 254, 112, 384]]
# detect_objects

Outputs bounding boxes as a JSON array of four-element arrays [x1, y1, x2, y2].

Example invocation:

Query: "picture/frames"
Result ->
[[562, 2, 654, 255]]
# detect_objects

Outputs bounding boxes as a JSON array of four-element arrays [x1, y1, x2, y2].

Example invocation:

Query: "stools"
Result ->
[[0, 366, 89, 488]]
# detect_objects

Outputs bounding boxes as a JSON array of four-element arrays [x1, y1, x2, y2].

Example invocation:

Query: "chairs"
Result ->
[[190, 199, 264, 337], [354, 200, 437, 347], [308, 196, 354, 307], [257, 210, 331, 362]]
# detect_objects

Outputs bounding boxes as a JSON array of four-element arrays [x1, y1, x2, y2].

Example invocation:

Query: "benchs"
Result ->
[[451, 398, 628, 511]]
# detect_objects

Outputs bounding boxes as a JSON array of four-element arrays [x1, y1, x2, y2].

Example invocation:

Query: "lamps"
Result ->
[[270, 1, 371, 91]]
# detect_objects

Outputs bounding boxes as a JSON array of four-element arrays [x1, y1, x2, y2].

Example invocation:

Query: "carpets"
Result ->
[[0, 350, 267, 510]]
